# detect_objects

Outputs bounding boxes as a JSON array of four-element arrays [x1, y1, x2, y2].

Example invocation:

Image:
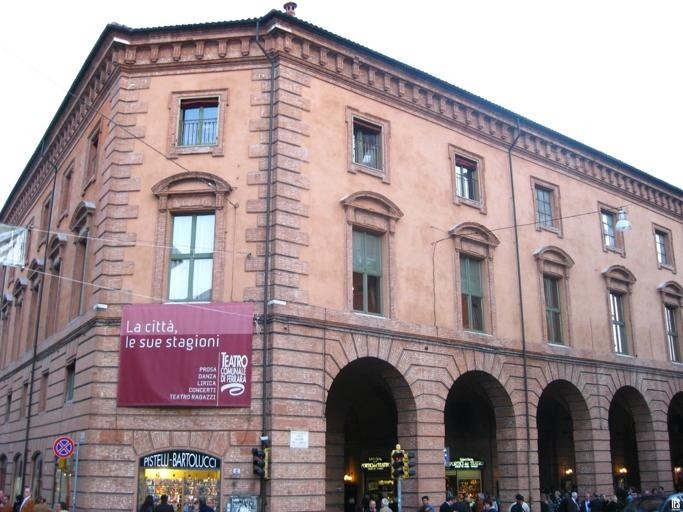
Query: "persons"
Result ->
[[0, 487, 48, 512], [362, 492, 398, 511], [155, 495, 174, 512], [140, 496, 154, 512], [439, 493, 530, 511], [540, 486, 663, 511], [198, 496, 214, 512], [420, 496, 435, 510]]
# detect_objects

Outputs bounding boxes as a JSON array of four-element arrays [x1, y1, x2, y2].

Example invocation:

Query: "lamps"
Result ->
[[615, 207, 630, 233]]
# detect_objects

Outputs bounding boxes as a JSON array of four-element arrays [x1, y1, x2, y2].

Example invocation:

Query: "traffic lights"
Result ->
[[390, 449, 416, 480], [251, 447, 271, 479]]
